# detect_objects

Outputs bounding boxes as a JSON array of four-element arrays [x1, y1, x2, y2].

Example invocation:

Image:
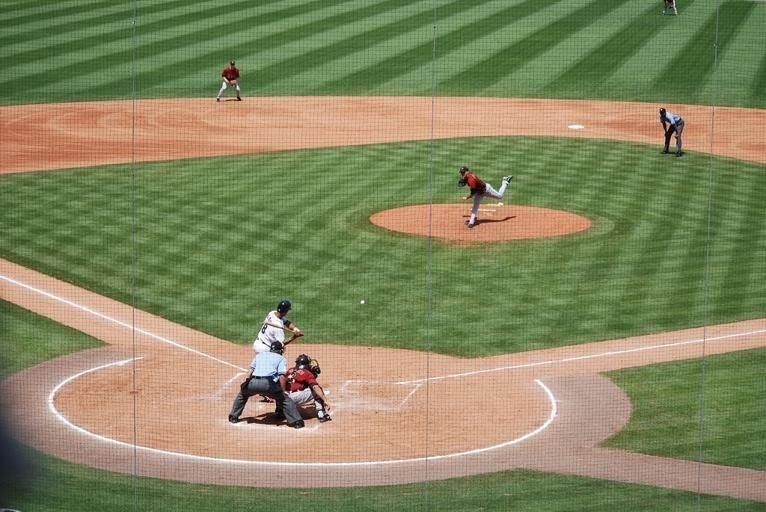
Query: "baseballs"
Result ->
[[361, 300, 364, 304]]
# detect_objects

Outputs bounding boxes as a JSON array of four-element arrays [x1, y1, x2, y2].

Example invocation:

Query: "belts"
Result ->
[[292, 388, 303, 392], [251, 375, 266, 379]]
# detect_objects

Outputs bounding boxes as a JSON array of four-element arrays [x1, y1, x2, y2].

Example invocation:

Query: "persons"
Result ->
[[216, 61, 241, 102], [228, 343, 305, 428], [658, 107, 684, 157], [458, 166, 514, 228], [285, 354, 330, 422], [662, 0, 677, 16], [253, 301, 304, 403]]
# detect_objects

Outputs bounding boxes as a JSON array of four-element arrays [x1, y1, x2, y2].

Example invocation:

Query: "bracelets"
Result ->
[[292, 327, 301, 333]]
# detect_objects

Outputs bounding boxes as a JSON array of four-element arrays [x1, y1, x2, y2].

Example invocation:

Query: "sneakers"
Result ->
[[503, 175, 512, 183], [294, 421, 304, 428], [228, 416, 237, 423]]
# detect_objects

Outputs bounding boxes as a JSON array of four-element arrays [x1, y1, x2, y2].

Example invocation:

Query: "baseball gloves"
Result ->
[[309, 359, 320, 374], [459, 179, 466, 187]]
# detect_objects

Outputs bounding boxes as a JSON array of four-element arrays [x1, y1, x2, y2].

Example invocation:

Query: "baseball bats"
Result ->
[[265, 322, 294, 332]]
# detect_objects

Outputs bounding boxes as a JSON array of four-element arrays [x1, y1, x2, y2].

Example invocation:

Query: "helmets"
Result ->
[[277, 300, 292, 313], [297, 355, 308, 369], [460, 166, 469, 173], [271, 341, 282, 350]]
